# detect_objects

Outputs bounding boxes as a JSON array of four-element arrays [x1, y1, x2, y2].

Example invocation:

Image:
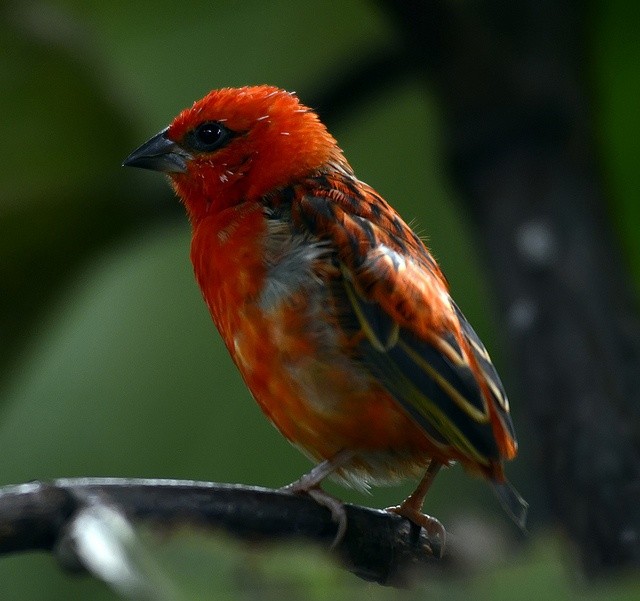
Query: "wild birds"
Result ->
[[123, 85, 527, 558]]
